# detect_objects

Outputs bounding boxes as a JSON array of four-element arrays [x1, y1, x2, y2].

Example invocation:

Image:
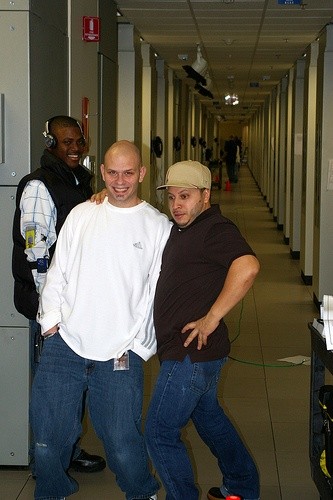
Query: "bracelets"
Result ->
[[44, 331, 56, 340]]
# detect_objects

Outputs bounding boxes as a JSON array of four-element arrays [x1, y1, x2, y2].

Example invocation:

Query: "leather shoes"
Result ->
[[73, 450, 106, 473]]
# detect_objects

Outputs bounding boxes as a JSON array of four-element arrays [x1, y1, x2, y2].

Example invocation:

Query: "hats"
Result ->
[[156, 159, 211, 190]]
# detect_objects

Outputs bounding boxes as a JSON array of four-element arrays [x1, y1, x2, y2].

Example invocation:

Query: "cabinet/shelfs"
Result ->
[[309, 294, 333, 500]]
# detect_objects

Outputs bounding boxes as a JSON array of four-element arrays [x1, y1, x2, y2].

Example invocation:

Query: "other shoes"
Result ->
[[206, 485, 226, 500]]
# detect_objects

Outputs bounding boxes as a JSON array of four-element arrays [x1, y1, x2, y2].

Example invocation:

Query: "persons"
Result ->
[[11, 116, 106, 473], [221, 135, 243, 183], [30, 140, 174, 500], [90, 160, 261, 500]]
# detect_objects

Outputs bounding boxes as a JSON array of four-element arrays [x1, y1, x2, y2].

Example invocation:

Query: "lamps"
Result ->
[[183, 44, 213, 99], [225, 75, 239, 106]]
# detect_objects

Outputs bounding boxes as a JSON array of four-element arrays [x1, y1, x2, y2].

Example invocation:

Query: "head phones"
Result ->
[[42, 117, 86, 149]]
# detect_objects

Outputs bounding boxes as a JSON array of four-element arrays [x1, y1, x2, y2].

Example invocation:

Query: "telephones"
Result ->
[[152, 136, 206, 158]]
